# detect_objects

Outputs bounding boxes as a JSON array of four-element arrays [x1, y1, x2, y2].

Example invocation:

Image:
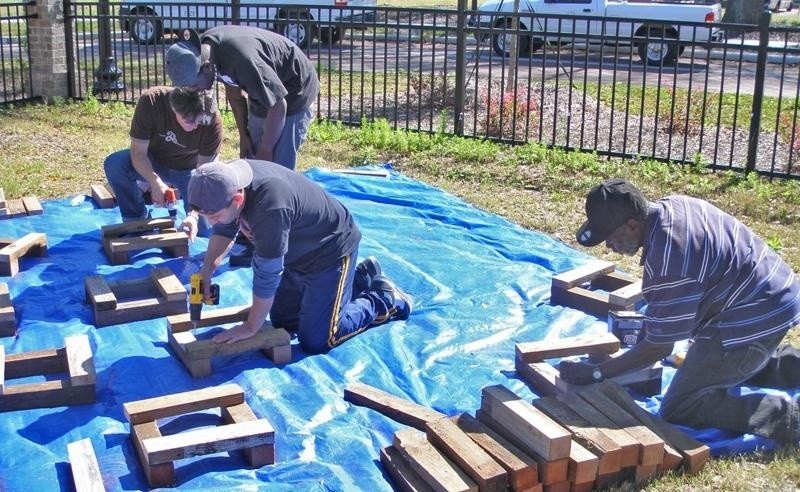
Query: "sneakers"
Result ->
[[357, 256, 381, 278], [369, 275, 413, 315], [229, 230, 254, 268]]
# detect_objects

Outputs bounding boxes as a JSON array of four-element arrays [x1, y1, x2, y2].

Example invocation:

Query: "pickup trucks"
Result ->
[[118, 0, 377, 50], [472, 0, 724, 67]]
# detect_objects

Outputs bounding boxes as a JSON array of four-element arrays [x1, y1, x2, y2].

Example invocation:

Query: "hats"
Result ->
[[576, 179, 646, 248], [164, 28, 201, 87], [186, 158, 252, 211]]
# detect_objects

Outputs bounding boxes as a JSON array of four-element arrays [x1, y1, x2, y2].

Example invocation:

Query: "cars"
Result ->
[[767, 0, 793, 13]]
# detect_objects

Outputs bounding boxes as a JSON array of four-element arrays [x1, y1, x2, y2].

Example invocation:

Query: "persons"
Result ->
[[164, 24, 320, 245], [190, 159, 413, 353], [559, 177, 800, 443], [105, 87, 224, 244]]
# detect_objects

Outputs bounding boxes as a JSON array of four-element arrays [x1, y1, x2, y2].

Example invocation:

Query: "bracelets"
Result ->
[[187, 214, 198, 221]]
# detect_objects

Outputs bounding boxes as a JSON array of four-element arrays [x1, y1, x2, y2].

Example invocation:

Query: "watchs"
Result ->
[[591, 364, 604, 383]]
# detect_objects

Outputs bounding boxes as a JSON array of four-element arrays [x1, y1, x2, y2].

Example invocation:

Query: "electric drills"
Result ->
[[144, 188, 178, 234], [190, 274, 220, 337]]
